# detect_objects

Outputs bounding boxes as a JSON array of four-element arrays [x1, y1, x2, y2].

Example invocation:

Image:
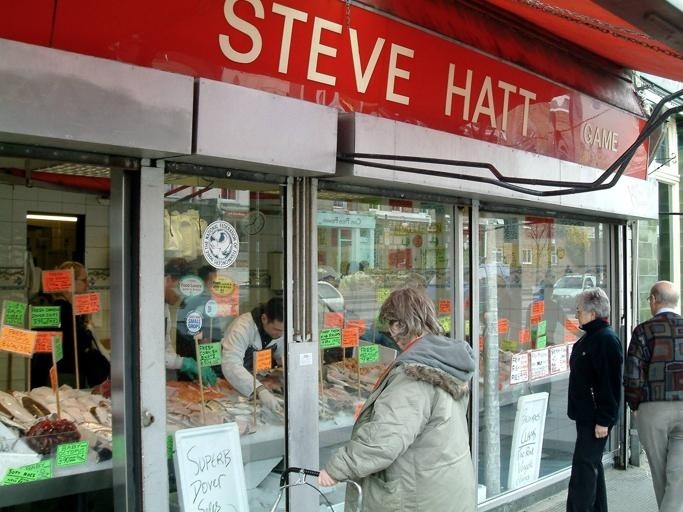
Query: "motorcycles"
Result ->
[[531, 279, 544, 303]]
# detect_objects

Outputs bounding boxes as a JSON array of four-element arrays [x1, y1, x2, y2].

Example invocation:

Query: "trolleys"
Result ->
[[267, 465, 363, 512]]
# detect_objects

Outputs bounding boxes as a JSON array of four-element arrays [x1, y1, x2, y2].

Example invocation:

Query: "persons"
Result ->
[[566, 289, 623, 511], [624, 280, 682, 512], [345, 260, 375, 288], [476, 276, 584, 350], [165, 259, 226, 385], [315, 288, 481, 511], [219, 297, 287, 411], [33, 259, 111, 390]]
